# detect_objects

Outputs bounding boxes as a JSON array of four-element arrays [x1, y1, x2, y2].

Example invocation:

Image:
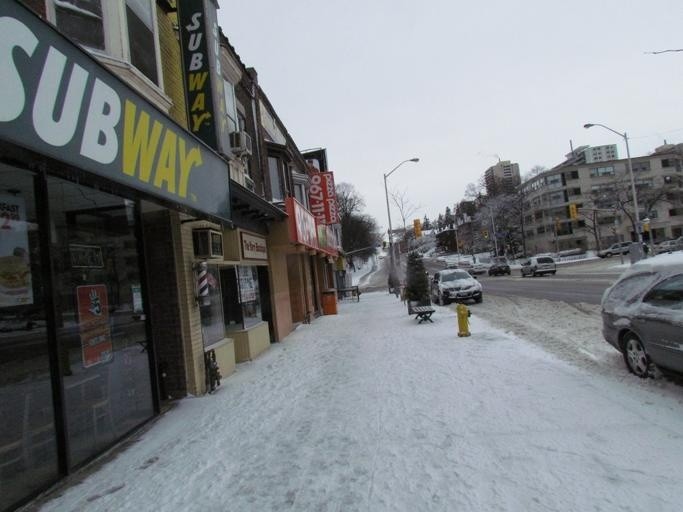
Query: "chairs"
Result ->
[[0, 367, 116, 488]]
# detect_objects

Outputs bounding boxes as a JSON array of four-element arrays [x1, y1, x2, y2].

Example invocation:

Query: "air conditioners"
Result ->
[[232, 129, 253, 157]]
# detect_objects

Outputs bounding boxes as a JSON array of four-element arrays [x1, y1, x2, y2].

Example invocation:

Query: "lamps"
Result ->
[[296, 245, 337, 261]]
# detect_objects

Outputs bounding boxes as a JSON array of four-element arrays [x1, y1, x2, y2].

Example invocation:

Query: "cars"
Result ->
[[487, 263, 513, 276], [468, 263, 487, 275], [600, 248, 683, 381], [649, 236, 683, 253]]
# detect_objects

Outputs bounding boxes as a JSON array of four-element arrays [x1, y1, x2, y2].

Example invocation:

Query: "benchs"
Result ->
[[411, 305, 436, 324]]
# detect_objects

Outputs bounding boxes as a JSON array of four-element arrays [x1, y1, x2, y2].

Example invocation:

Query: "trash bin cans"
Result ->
[[322, 289, 337, 315]]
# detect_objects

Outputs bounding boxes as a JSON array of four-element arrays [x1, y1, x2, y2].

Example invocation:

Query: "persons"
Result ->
[[642, 241, 649, 257]]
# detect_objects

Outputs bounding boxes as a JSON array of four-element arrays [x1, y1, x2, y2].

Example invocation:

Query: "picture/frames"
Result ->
[[68, 244, 104, 269], [240, 231, 269, 260], [75, 284, 115, 370]]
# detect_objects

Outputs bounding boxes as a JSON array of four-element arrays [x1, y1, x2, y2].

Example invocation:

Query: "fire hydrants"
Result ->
[[455, 300, 472, 338]]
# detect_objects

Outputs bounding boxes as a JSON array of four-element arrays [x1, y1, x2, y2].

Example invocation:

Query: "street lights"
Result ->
[[583, 122, 644, 244], [383, 157, 420, 268]]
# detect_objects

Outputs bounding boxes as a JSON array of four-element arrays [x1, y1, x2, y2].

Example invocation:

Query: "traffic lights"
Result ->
[[482, 229, 489, 238]]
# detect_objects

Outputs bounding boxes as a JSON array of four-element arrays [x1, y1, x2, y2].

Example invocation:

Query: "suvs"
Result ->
[[429, 268, 484, 307], [519, 254, 558, 278], [596, 240, 634, 259]]
